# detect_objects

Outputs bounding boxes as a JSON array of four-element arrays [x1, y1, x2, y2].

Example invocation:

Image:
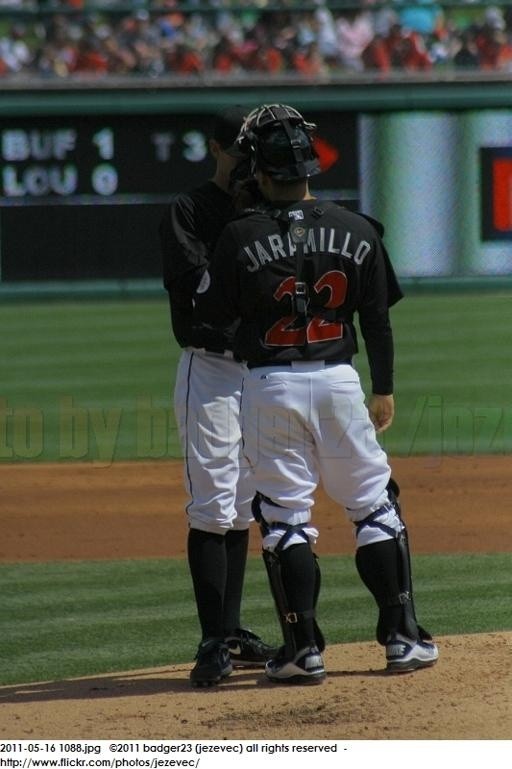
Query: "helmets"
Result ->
[[232, 105, 322, 184]]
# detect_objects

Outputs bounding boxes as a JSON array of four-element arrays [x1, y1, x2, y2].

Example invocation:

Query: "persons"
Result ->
[[0, 0, 511, 77], [187, 103, 443, 685], [162, 105, 282, 687]]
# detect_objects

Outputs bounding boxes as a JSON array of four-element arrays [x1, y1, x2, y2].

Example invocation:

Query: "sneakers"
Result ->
[[190, 638, 232, 688], [385, 631, 439, 672], [223, 626, 276, 667], [265, 640, 325, 684]]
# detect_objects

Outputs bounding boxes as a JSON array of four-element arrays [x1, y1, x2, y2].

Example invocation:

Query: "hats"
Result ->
[[213, 106, 252, 158]]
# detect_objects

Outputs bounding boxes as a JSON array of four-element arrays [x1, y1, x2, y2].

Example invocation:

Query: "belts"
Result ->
[[248, 356, 352, 370], [204, 346, 243, 362]]
